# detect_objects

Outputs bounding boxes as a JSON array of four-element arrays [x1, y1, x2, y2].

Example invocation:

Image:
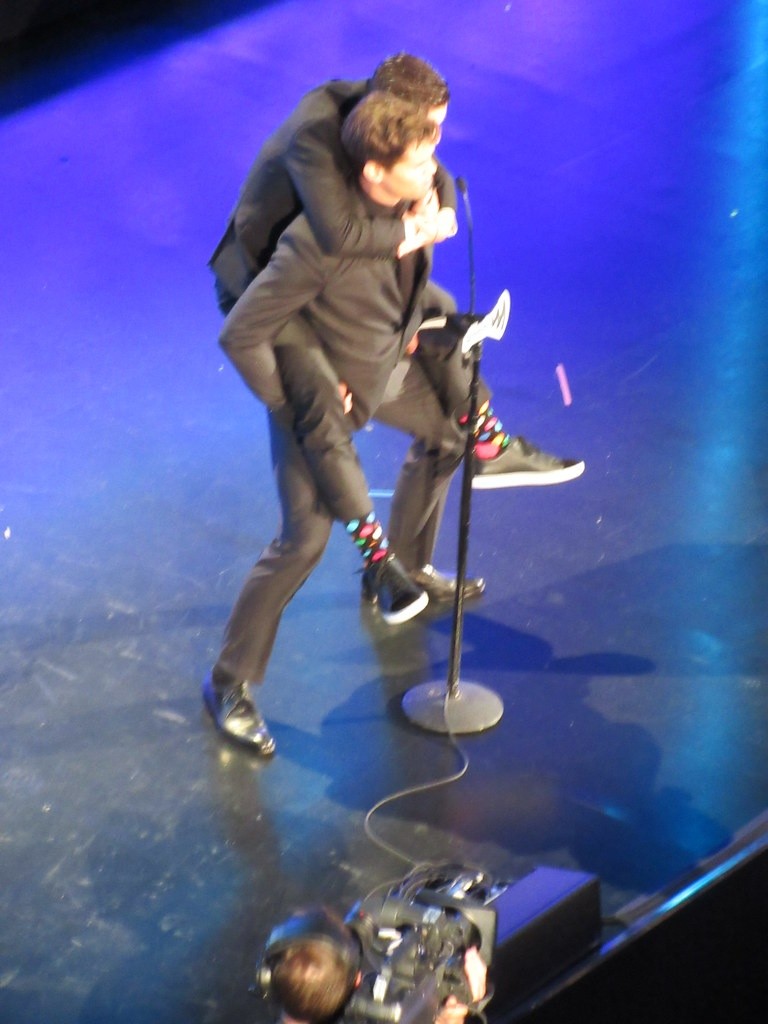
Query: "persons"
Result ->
[[269, 906, 485, 1024], [207, 52, 585, 623], [203, 91, 485, 757]]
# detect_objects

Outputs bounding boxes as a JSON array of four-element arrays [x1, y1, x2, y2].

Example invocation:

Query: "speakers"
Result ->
[[486, 869, 604, 1013]]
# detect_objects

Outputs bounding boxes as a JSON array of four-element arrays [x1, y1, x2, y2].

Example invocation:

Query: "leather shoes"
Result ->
[[404, 563, 486, 602], [206, 664, 276, 754]]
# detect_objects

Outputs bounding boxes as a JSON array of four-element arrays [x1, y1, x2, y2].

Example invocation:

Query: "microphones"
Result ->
[[455, 177, 476, 317]]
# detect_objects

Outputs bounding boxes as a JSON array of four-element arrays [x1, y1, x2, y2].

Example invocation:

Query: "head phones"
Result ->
[[250, 916, 359, 1002]]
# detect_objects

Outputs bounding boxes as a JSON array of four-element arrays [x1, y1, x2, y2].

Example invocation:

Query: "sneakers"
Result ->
[[363, 550, 429, 625], [470, 435, 586, 490]]
